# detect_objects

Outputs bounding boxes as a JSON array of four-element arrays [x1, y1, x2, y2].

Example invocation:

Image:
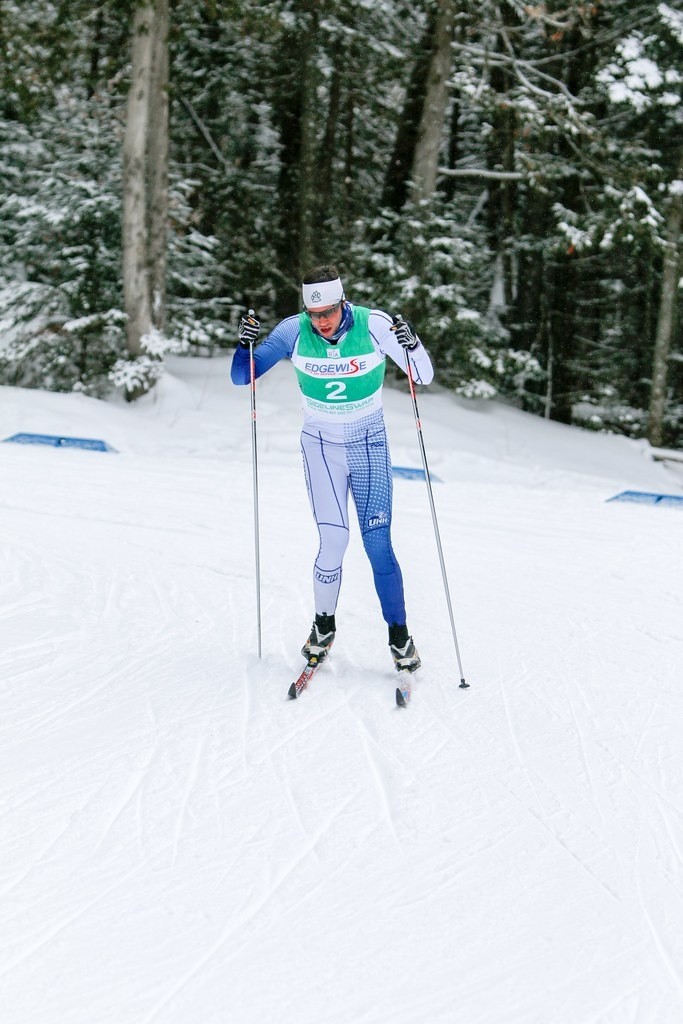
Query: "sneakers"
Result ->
[[301, 614, 336, 663], [388, 625, 421, 673]]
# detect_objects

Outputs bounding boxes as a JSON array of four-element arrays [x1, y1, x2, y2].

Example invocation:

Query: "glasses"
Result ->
[[303, 298, 343, 320]]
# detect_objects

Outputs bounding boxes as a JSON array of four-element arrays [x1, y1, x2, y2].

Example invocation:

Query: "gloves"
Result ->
[[239, 314, 261, 349], [391, 314, 418, 349]]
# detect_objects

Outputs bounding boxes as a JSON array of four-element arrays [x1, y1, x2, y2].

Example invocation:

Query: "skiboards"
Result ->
[[287, 615, 422, 707]]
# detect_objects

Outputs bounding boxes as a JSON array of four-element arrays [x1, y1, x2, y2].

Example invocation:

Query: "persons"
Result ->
[[230, 265, 435, 675]]
